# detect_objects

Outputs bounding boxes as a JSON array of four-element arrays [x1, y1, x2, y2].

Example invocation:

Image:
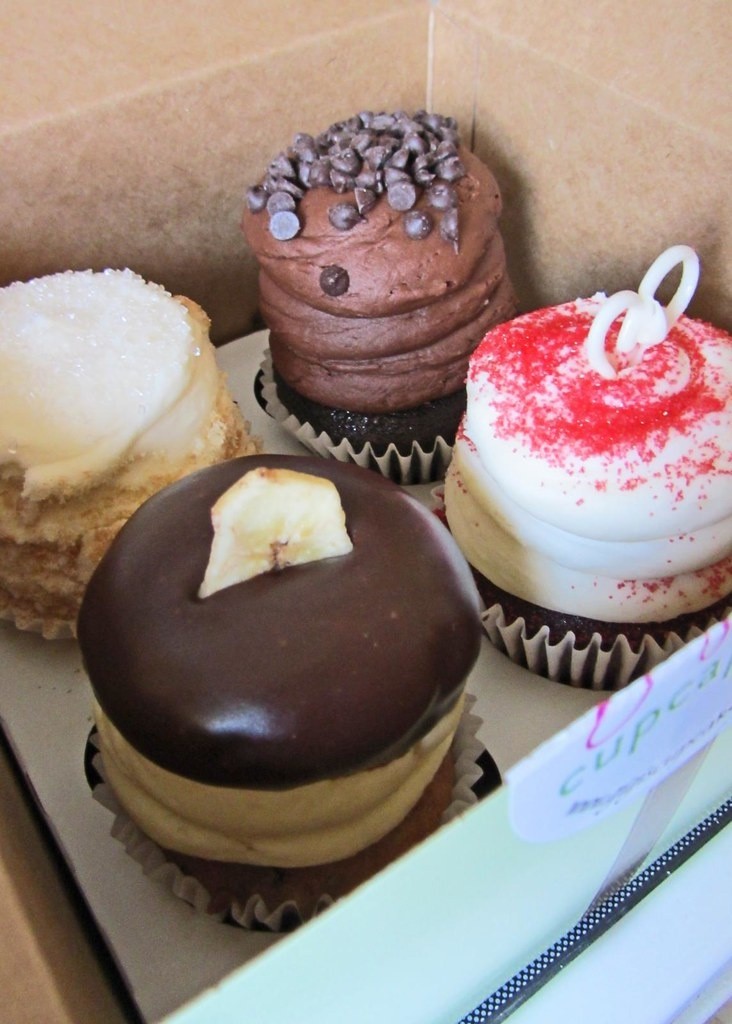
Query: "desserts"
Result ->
[[1, 111, 732, 934]]
